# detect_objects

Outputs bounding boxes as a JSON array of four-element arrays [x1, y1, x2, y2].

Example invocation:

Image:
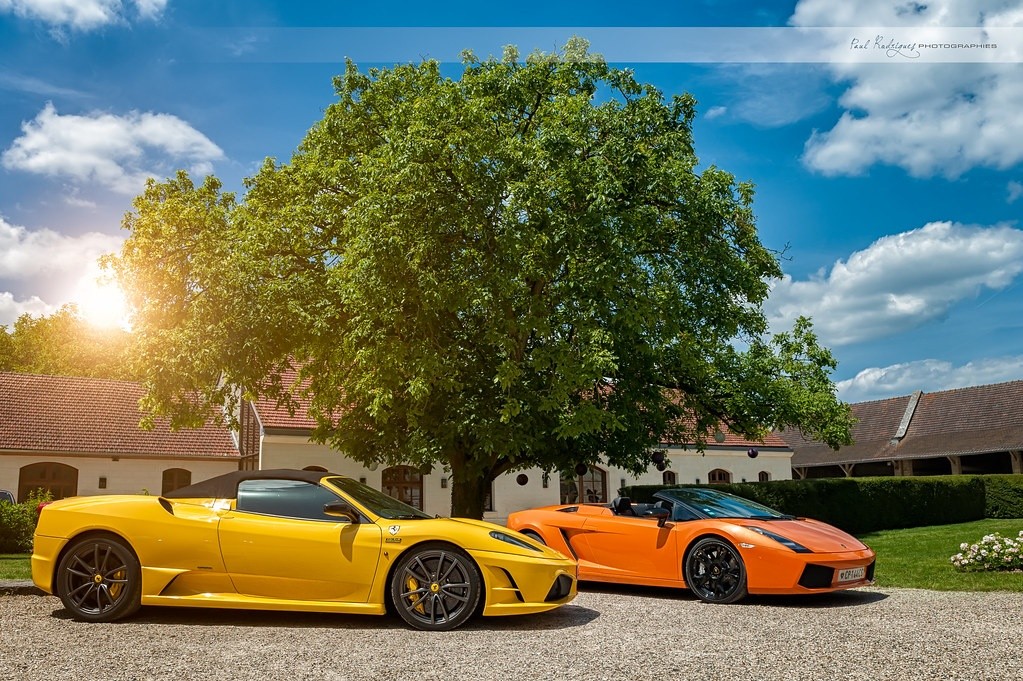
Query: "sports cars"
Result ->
[[31, 468, 580, 632], [505, 487, 875, 604]]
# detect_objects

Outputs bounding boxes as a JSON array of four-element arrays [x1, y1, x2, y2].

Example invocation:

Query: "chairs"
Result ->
[[653, 501, 672, 517], [611, 497, 636, 516]]
